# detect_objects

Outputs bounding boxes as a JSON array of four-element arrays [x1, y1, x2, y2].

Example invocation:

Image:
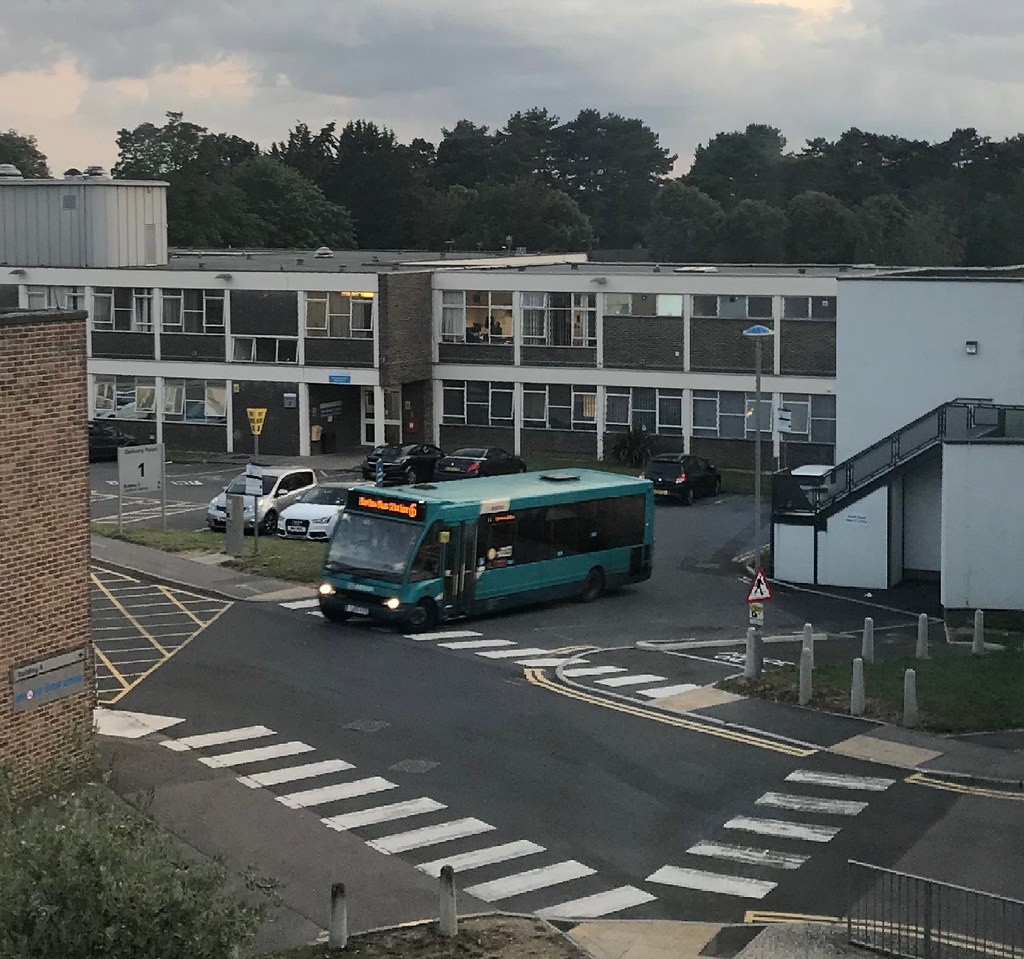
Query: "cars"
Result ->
[[638, 452, 723, 506], [275, 480, 375, 543], [361, 443, 448, 484], [98, 389, 224, 423], [434, 444, 528, 485], [87, 419, 140, 462]]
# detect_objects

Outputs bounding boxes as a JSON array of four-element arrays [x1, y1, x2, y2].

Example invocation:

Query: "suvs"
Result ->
[[204, 466, 318, 536]]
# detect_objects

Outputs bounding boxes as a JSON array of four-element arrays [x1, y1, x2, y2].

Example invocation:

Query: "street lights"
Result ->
[[742, 325, 777, 571]]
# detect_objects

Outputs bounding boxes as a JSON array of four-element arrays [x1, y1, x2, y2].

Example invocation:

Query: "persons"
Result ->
[[483, 322, 502, 341]]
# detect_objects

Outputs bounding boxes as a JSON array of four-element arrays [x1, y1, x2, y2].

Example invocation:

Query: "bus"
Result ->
[[316, 467, 656, 634]]
